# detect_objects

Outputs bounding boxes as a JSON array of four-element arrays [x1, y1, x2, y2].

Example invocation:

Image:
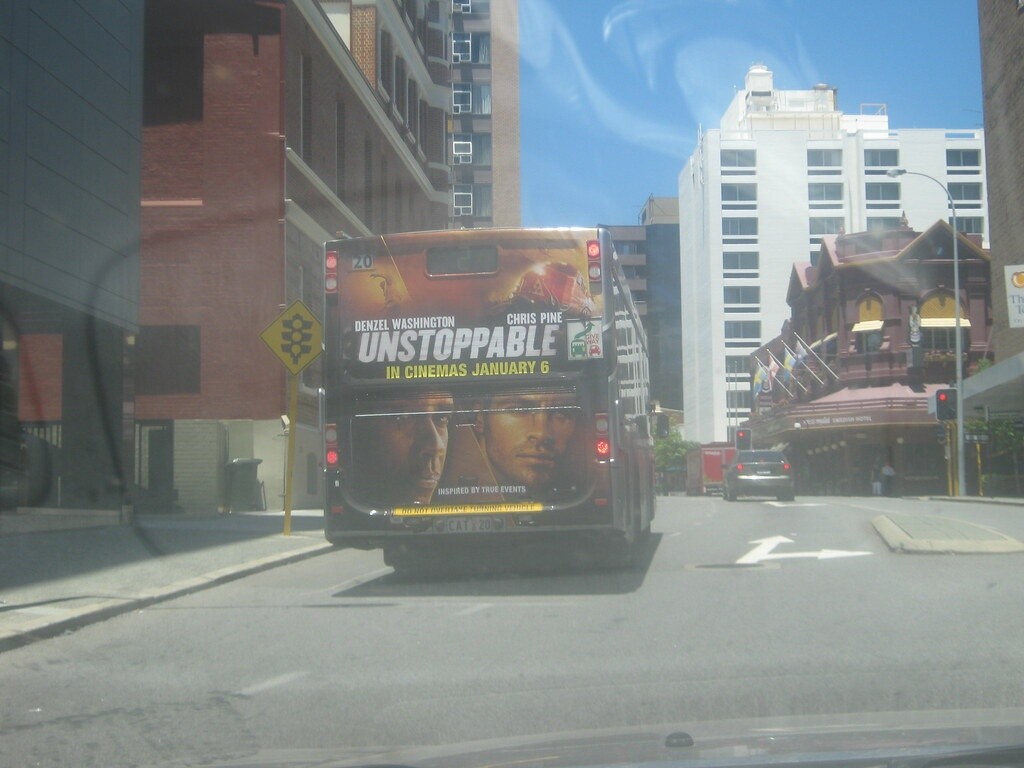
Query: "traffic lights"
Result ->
[[937, 388, 958, 420], [734, 429, 753, 451]]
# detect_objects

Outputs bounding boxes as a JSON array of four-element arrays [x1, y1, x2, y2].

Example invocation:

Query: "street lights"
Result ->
[[886, 167, 967, 495]]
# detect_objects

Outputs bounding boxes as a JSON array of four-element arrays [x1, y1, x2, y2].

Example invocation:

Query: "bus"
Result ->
[[320, 225, 671, 577]]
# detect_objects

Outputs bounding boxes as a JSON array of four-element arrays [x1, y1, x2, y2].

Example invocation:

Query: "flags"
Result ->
[[751, 341, 808, 398]]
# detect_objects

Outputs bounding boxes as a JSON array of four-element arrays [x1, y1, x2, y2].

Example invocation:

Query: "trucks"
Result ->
[[685, 446, 738, 495]]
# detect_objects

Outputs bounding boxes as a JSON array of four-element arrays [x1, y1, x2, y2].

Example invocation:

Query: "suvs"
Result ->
[[719, 450, 799, 502]]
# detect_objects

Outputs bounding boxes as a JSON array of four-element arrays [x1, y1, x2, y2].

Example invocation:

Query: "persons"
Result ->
[[339, 392, 455, 510], [868, 460, 897, 498], [468, 371, 586, 497]]
